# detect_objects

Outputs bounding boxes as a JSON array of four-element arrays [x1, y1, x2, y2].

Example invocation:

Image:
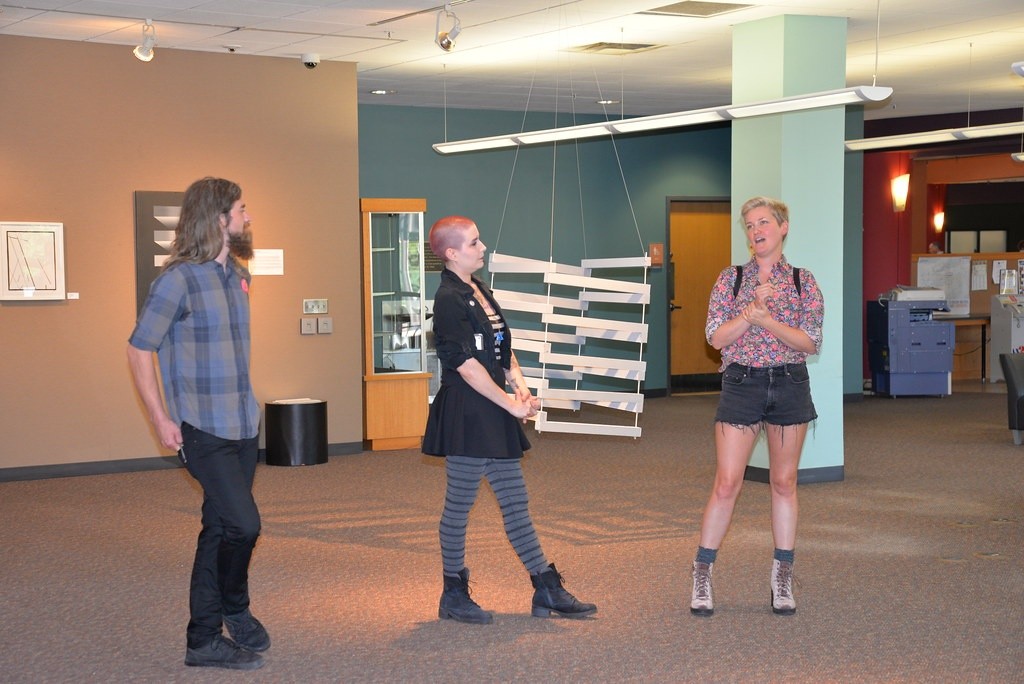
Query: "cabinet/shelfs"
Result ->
[[359, 198, 433, 451]]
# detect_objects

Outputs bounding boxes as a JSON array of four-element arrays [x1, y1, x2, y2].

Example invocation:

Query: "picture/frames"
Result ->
[[0, 221, 66, 301]]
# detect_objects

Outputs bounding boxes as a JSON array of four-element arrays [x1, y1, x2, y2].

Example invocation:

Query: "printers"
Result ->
[[866, 285, 955, 400]]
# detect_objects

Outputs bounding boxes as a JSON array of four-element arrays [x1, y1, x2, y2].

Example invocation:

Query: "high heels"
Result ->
[[769, 558, 796, 615], [690, 559, 713, 616]]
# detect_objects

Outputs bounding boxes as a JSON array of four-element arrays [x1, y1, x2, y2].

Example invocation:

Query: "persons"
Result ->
[[929, 243, 942, 254], [690, 196, 823, 616], [421, 217, 597, 625], [126, 176, 271, 669]]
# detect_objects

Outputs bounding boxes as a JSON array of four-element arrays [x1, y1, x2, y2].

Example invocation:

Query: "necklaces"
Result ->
[[467, 281, 504, 340]]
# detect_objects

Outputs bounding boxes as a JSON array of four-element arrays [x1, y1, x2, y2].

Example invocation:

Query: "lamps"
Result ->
[[434, 5, 462, 53], [132, 19, 159, 62], [432, 0, 893, 155], [1011, 61, 1024, 163], [844, 42, 1024, 152]]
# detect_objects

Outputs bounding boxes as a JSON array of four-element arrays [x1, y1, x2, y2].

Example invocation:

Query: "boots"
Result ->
[[438, 567, 494, 624], [532, 562, 597, 617]]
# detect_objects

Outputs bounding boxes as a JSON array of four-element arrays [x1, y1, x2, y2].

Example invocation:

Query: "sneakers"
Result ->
[[184, 630, 265, 670], [221, 611, 271, 651]]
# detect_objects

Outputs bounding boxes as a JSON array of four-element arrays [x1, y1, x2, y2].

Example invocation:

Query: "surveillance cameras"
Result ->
[[300, 54, 321, 69]]
